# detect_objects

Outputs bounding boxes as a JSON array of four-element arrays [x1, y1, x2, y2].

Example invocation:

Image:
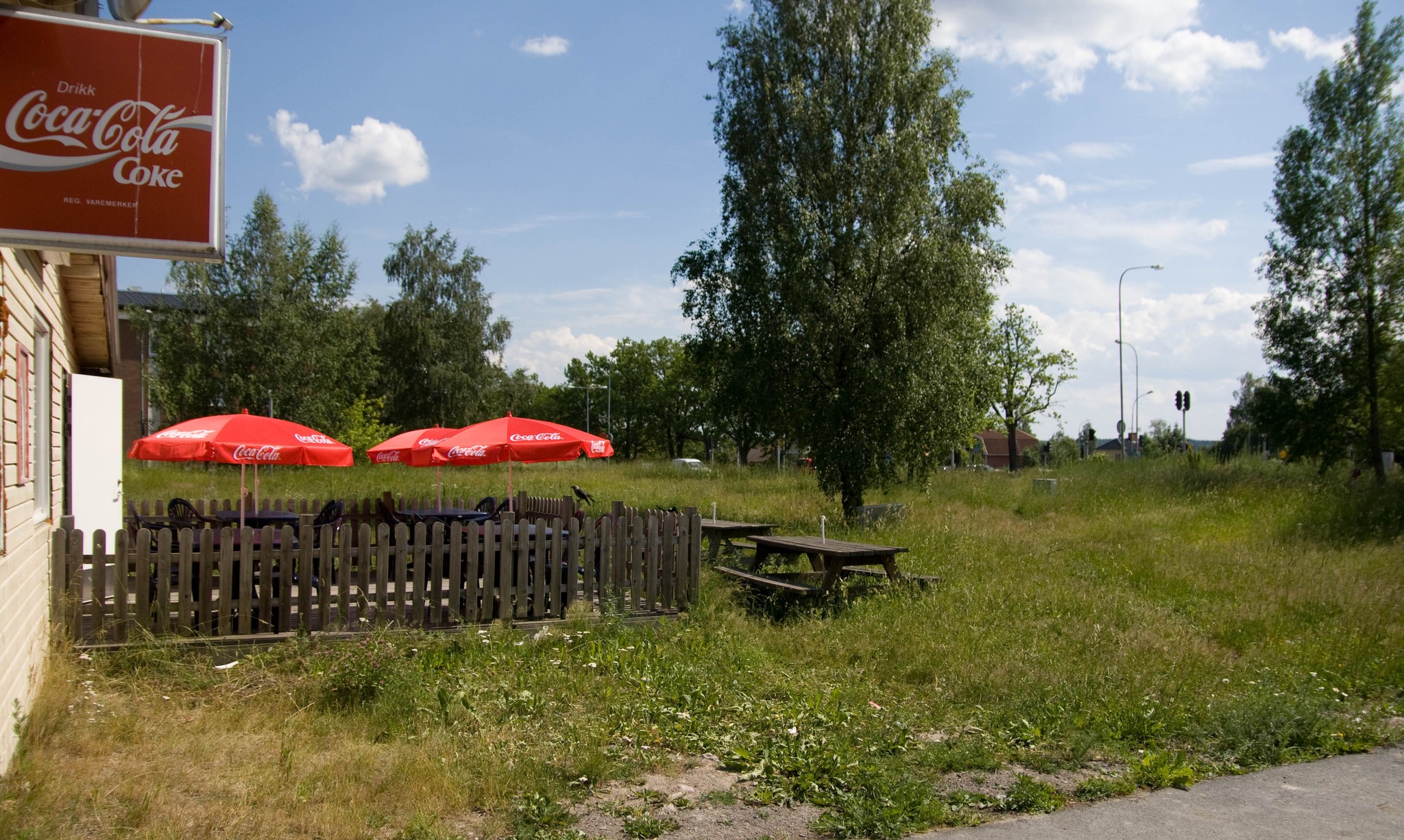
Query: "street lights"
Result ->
[[1113, 265, 1166, 460], [568, 385, 608, 433]]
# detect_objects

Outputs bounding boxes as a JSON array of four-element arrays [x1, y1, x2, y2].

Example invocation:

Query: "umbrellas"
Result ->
[[365, 411, 614, 512], [128, 409, 354, 528]]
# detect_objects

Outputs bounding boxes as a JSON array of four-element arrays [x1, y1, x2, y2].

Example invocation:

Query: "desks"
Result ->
[[701, 517, 780, 565], [394, 508, 489, 521], [745, 534, 909, 624], [191, 528, 300, 599], [215, 510, 300, 521], [462, 526, 569, 537]]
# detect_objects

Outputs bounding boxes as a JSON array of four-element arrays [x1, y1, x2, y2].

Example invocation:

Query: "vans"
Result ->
[[672, 457, 712, 478]]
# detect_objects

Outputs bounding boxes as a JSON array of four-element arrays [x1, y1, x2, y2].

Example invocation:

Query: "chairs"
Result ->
[[127, 496, 612, 630]]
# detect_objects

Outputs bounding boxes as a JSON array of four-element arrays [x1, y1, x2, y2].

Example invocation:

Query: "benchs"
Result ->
[[712, 541, 938, 596]]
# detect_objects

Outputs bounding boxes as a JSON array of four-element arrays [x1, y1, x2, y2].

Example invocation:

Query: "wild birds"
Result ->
[[656, 505, 678, 515], [571, 485, 595, 506]]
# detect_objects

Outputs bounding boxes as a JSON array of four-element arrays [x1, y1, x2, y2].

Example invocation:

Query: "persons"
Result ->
[[1279, 448, 1287, 461], [1353, 467, 1359, 480], [1265, 448, 1271, 460], [1317, 461, 1328, 478]]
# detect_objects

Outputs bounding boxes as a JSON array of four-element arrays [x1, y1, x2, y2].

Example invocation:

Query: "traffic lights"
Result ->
[[1175, 391, 1182, 410], [1183, 391, 1190, 410]]
